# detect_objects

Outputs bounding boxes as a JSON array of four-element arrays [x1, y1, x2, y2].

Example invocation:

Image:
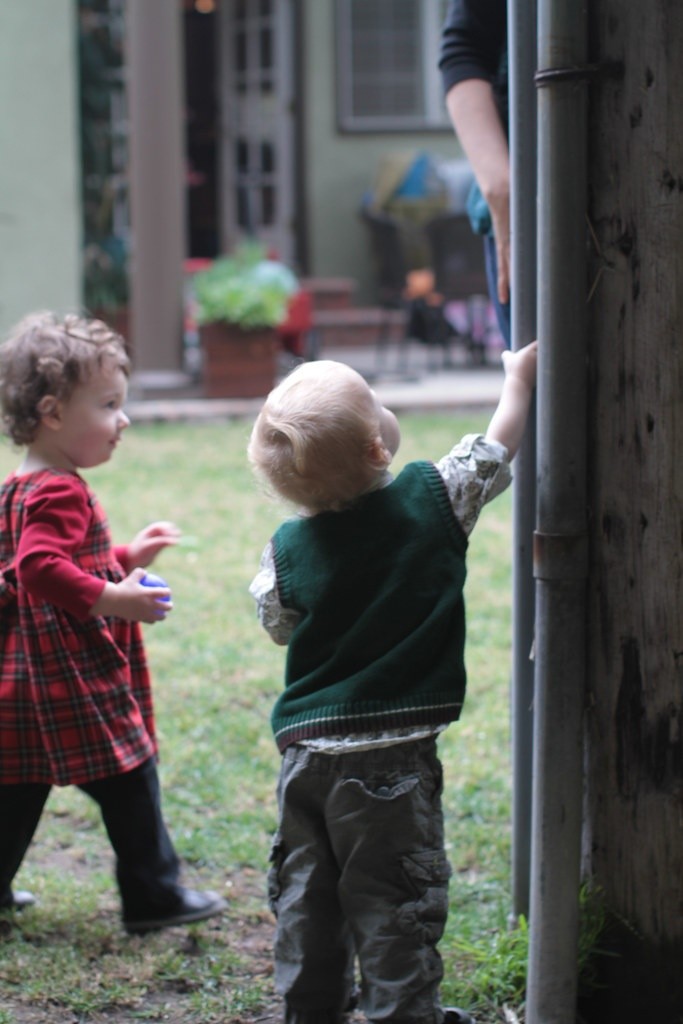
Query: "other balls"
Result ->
[[139, 572, 170, 614]]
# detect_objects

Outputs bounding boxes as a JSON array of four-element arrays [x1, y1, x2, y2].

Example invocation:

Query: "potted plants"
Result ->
[[192, 237, 299, 401], [75, 241, 132, 368]]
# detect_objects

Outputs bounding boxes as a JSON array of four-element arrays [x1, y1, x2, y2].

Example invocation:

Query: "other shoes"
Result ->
[[0, 891, 40, 917], [124, 887, 227, 929], [441, 1006, 475, 1024]]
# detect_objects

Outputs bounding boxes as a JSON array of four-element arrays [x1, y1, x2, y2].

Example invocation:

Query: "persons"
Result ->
[[248, 340, 538, 1024], [436, 0, 511, 352], [0, 312, 228, 936]]
